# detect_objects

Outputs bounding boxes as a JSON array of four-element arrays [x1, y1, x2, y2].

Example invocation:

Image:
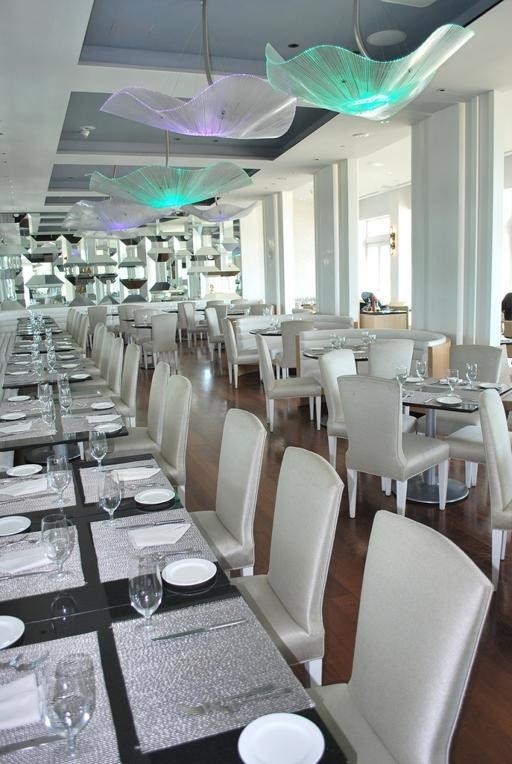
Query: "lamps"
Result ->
[[63, 164, 174, 233], [98, 1, 296, 140], [90, 130, 251, 208], [180, 197, 258, 221], [266, 0, 473, 120], [390, 238, 395, 249], [390, 226, 395, 240]]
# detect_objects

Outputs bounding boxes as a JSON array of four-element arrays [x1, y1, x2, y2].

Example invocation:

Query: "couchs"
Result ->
[[296, 330, 451, 378], [234, 315, 359, 376]]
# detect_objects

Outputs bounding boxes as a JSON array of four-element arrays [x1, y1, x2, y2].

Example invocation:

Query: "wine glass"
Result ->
[[67, 519, 75, 552], [293, 314, 304, 321], [41, 400, 56, 435], [328, 331, 336, 350], [448, 369, 459, 396], [98, 471, 121, 528], [128, 562, 163, 628], [286, 314, 292, 321], [466, 363, 477, 390], [31, 335, 41, 377], [369, 327, 376, 343], [263, 306, 273, 331], [42, 514, 71, 583], [89, 432, 108, 470], [30, 313, 42, 331], [337, 336, 345, 349], [47, 455, 69, 506], [42, 653, 97, 762], [142, 312, 147, 326], [274, 318, 278, 331], [416, 360, 426, 385], [396, 367, 407, 391], [59, 389, 72, 417], [49, 592, 79, 625], [45, 327, 56, 373], [57, 374, 70, 407], [38, 384, 49, 399]]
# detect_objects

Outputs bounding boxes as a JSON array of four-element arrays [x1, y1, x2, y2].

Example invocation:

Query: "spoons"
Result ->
[[0, 653, 23, 669], [26, 539, 39, 543], [209, 688, 292, 713]]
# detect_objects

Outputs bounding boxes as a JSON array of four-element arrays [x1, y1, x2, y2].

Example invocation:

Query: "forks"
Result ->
[[8, 534, 29, 543], [15, 651, 49, 673], [179, 683, 275, 714]]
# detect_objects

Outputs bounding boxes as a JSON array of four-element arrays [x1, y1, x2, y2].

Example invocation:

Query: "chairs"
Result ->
[[478, 389, 512, 592], [83, 327, 107, 376], [320, 350, 417, 473], [417, 345, 507, 489], [94, 376, 191, 509], [208, 301, 222, 304], [87, 306, 105, 350], [205, 309, 228, 363], [67, 310, 76, 338], [71, 312, 78, 339], [73, 338, 123, 401], [303, 510, 494, 764], [223, 319, 261, 388], [369, 340, 413, 380], [183, 303, 209, 348], [251, 306, 273, 318], [229, 448, 344, 687], [72, 314, 83, 347], [74, 316, 89, 358], [185, 408, 265, 577], [81, 322, 104, 365], [106, 344, 141, 428], [85, 362, 171, 459], [207, 305, 227, 332], [68, 333, 114, 389], [231, 299, 248, 305], [143, 314, 178, 376], [275, 321, 313, 380], [134, 310, 160, 359], [118, 306, 144, 344], [64, 308, 72, 335], [175, 302, 194, 342]]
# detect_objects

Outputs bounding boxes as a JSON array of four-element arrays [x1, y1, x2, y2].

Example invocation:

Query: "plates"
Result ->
[[237, 712, 326, 764], [14, 361, 30, 364], [58, 355, 74, 358], [5, 464, 43, 476], [19, 324, 32, 331], [19, 345, 29, 347], [56, 342, 67, 344], [58, 346, 72, 348], [7, 396, 30, 401], [71, 373, 91, 379], [436, 397, 463, 404], [93, 423, 122, 433], [479, 383, 496, 389], [406, 377, 424, 382], [0, 615, 25, 649], [9, 371, 28, 375], [353, 351, 366, 353], [311, 348, 324, 350], [48, 324, 63, 332], [0, 515, 32, 536], [61, 364, 80, 369], [0, 412, 26, 420], [90, 402, 116, 410], [161, 557, 218, 586], [16, 354, 30, 356], [134, 488, 175, 505]]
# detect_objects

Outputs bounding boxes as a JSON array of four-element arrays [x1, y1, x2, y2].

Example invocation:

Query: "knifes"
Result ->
[[0, 430, 40, 438], [73, 394, 103, 398], [115, 519, 185, 529], [0, 569, 59, 580], [0, 492, 59, 505], [150, 618, 248, 641], [0, 734, 68, 756]]
